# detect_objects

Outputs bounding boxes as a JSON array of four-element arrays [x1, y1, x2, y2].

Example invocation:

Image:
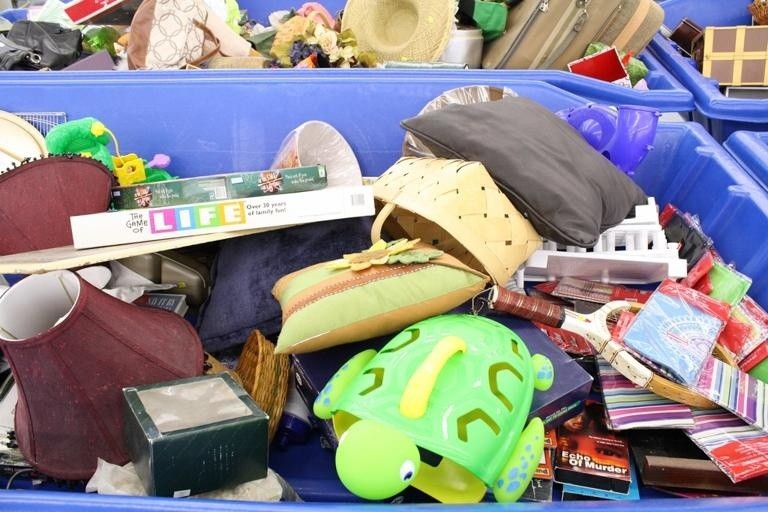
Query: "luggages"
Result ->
[[482, 1, 665, 72]]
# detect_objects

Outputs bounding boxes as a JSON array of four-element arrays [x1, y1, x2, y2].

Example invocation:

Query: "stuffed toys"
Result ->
[[44, 117, 171, 187]]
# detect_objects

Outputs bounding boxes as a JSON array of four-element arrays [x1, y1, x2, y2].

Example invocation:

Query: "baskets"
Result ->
[[368, 155, 542, 292], [205, 327, 290, 440]]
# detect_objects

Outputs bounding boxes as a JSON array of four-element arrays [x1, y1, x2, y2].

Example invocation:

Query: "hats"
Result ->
[[341, 0, 459, 65]]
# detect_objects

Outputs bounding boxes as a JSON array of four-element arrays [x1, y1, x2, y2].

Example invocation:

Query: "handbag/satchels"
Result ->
[[128, 0, 220, 71], [271, 11, 333, 67], [0, 18, 82, 70]]
